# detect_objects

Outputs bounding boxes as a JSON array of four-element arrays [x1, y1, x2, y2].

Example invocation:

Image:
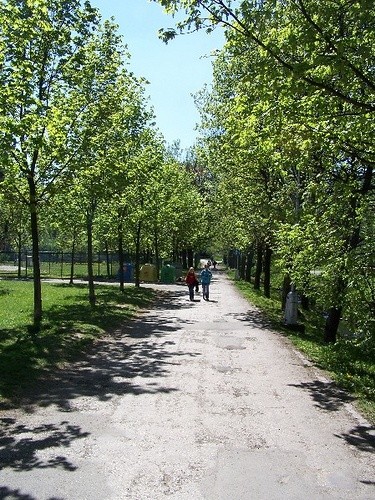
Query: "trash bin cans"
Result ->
[[160, 265, 176, 282], [285, 292, 298, 326], [116, 263, 133, 282], [139, 263, 157, 282]]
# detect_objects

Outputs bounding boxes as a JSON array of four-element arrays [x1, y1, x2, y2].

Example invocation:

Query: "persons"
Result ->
[[200, 264, 212, 301], [207, 261, 212, 269], [213, 261, 217, 268], [186, 267, 199, 300]]
